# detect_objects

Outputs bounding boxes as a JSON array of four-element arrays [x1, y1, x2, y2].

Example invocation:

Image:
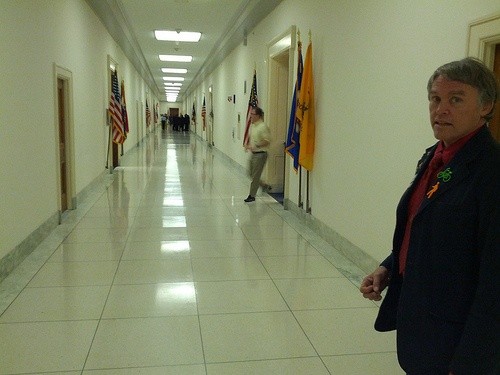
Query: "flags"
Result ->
[[295, 42, 315, 172], [201, 96, 207, 131], [109, 69, 126, 144], [285, 40, 304, 174], [120, 79, 129, 133], [146, 100, 152, 128], [243, 69, 259, 148]]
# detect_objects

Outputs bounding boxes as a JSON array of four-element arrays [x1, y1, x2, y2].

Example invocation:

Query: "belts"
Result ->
[[252, 151, 265, 153]]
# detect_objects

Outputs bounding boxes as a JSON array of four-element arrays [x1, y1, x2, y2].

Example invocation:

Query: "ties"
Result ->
[[399, 150, 442, 274]]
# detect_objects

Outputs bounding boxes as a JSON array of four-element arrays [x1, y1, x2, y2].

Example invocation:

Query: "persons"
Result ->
[[161, 113, 190, 132], [361, 57, 500, 375], [243, 106, 273, 202]]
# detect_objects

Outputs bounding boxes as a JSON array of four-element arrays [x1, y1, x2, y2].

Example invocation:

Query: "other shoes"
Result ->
[[244, 195, 255, 202]]
